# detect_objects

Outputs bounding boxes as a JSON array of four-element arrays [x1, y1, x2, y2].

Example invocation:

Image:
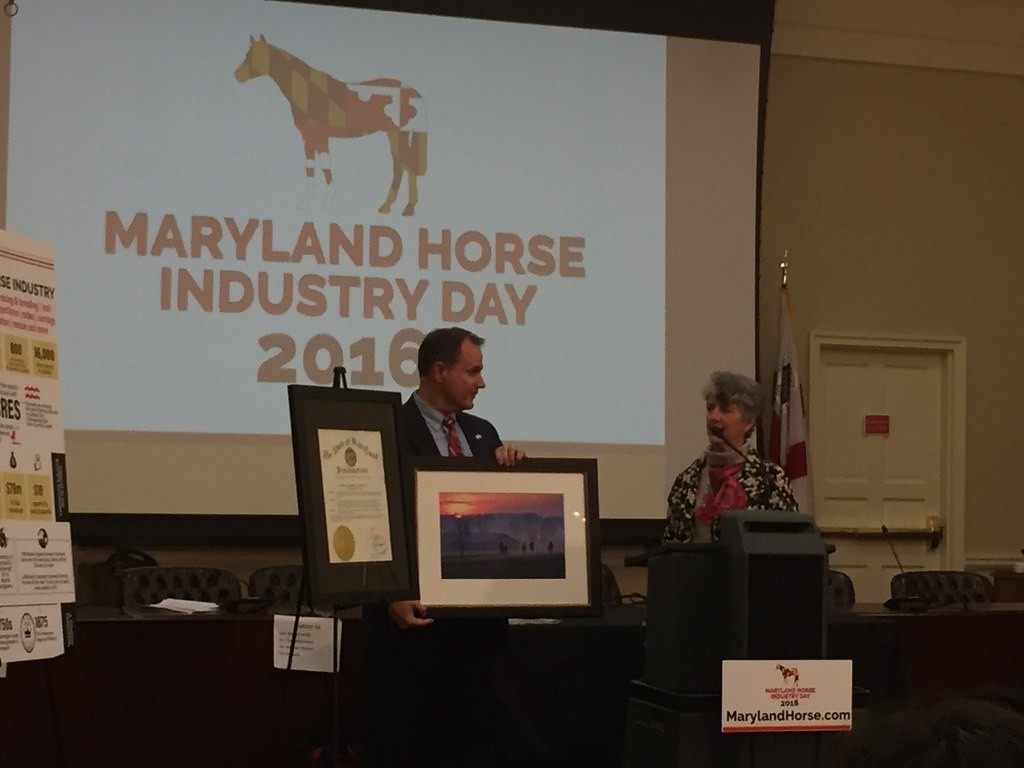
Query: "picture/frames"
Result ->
[[288, 383, 408, 601], [403, 456, 603, 620]]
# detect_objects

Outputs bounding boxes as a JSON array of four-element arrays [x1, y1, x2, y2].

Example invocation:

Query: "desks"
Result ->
[[64, 601, 1024, 768]]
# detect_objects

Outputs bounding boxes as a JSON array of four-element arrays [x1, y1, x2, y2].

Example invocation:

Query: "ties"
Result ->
[[442, 414, 464, 457]]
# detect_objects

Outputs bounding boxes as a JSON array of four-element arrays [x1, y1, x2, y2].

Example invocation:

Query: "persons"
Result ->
[[663, 370, 799, 543], [385, 327, 525, 628]]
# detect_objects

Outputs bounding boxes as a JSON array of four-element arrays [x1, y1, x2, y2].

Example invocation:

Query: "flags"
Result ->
[[768, 288, 811, 518]]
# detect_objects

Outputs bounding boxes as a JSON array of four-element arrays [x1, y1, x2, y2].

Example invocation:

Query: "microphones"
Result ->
[[879, 526, 929, 612], [711, 426, 794, 514]]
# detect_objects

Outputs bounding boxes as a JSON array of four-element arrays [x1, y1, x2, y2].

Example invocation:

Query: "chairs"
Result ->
[[891, 570, 988, 605], [249, 564, 305, 604], [117, 564, 241, 607]]
